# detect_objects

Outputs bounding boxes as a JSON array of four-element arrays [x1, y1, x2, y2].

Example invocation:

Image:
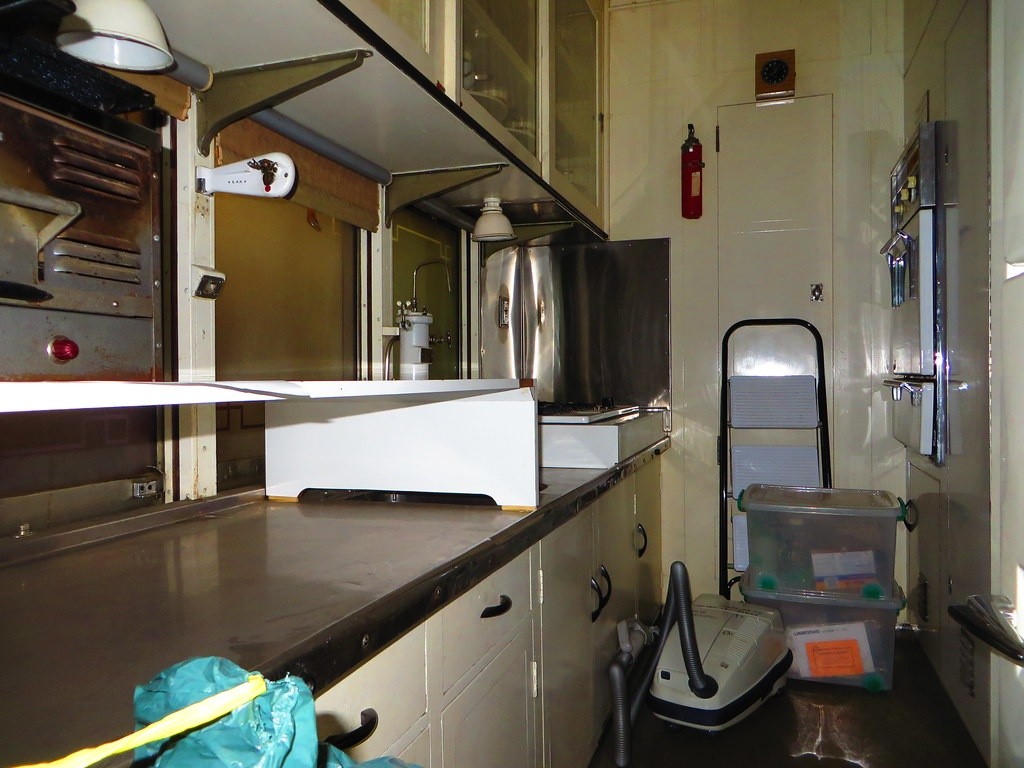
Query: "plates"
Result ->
[[464, 89, 536, 158]]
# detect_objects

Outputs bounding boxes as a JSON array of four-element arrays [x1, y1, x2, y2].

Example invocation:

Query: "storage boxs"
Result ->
[[738, 483, 905, 598], [740, 565, 907, 692]]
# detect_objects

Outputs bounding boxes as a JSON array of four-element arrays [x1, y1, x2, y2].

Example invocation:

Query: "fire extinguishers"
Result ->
[[680, 123, 704, 220]]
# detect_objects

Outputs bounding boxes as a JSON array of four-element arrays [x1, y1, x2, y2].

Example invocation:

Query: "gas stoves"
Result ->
[[537, 398, 641, 424]]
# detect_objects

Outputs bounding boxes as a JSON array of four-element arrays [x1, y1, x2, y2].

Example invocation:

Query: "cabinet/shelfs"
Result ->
[[445, 0, 604, 231], [315, 550, 527, 767], [635, 453, 661, 628], [540, 473, 635, 768]]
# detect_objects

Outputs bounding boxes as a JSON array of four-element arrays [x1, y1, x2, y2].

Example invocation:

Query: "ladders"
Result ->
[[717, 318, 832, 602]]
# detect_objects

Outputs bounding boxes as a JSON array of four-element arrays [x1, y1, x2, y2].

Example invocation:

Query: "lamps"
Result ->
[[472, 198, 517, 242], [55, 0, 173, 72]]
